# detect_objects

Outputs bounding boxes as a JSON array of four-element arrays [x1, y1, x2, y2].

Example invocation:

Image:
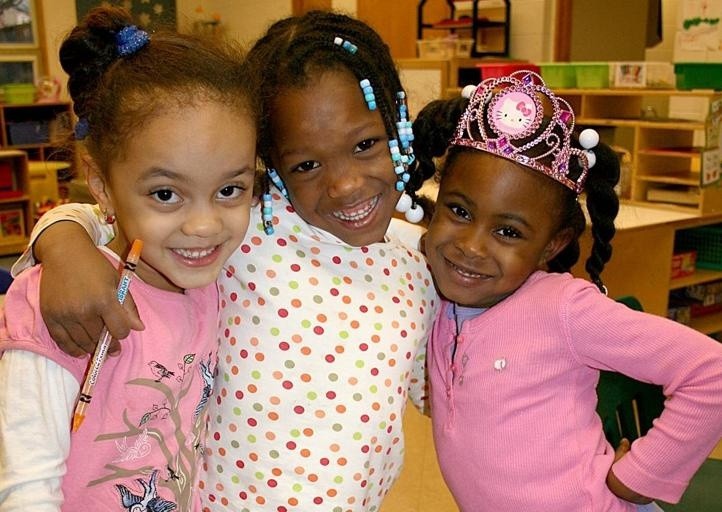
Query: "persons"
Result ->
[[9, 10, 441, 512], [0, 2, 264, 512], [407, 69, 722, 512]]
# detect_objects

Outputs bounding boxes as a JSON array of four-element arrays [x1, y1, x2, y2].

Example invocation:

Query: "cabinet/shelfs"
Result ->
[[0, 103, 87, 259], [444, 84, 721, 338], [414, 2, 512, 58]]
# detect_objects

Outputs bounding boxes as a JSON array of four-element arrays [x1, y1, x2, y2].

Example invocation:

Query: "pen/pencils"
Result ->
[[70, 238, 145, 434]]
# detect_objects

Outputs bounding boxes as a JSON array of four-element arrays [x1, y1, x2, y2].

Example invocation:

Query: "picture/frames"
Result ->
[[0, 12, 41, 85]]
